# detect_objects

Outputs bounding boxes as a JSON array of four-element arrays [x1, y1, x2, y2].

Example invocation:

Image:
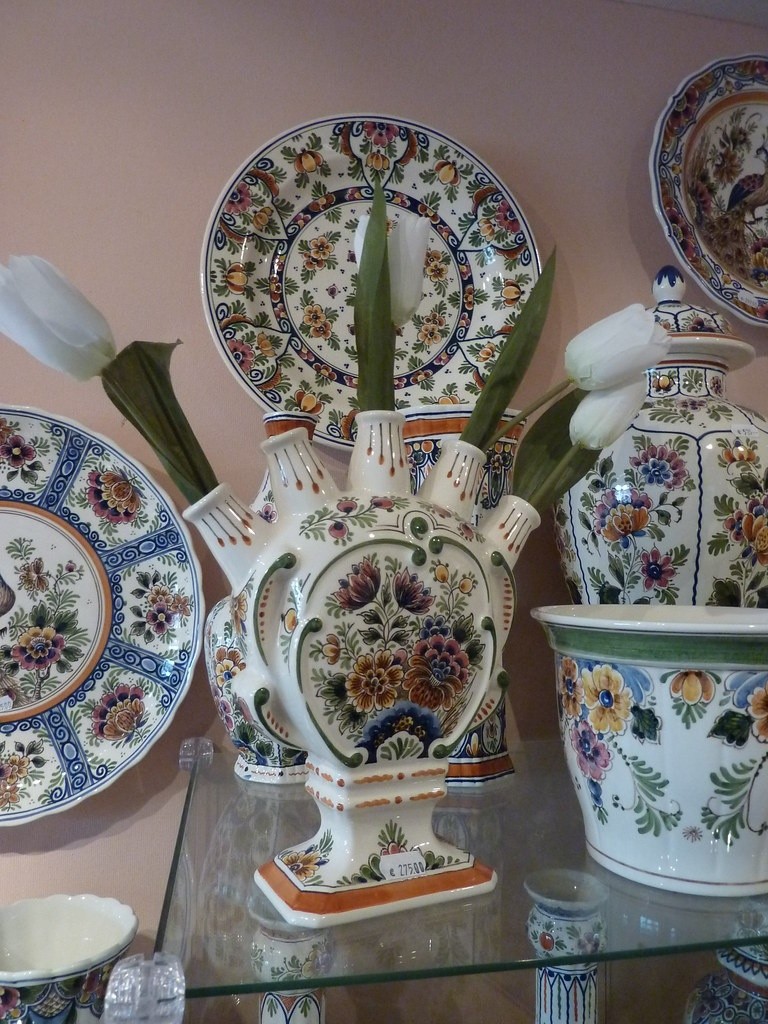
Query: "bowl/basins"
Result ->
[[533, 606, 768, 893]]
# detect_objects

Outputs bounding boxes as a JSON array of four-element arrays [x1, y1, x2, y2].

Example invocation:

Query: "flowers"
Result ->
[[0, 172, 674, 519]]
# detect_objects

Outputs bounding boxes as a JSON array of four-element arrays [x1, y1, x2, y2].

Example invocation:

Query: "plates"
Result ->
[[0, 404, 206, 826], [203, 113, 543, 452], [649, 55, 768, 328]]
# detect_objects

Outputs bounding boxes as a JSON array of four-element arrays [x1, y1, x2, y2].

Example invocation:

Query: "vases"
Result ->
[[0, 890, 140, 1024], [176, 408, 539, 931], [527, 604, 768, 898]]
